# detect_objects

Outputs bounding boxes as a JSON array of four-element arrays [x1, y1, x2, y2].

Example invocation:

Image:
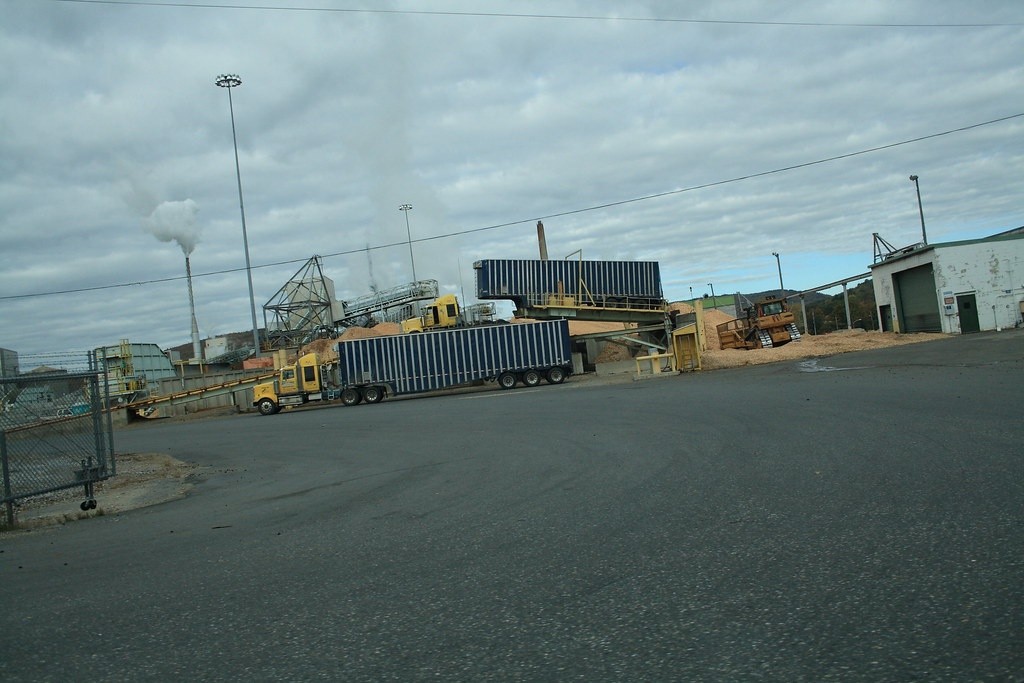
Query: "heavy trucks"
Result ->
[[250, 317, 573, 416], [400, 260, 663, 334]]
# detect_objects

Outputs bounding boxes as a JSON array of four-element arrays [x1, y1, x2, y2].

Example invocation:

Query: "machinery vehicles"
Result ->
[[716, 295, 801, 350]]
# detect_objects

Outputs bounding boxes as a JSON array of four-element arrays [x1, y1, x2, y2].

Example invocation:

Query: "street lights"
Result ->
[[771, 252, 785, 297], [909, 174, 929, 245], [214, 75, 261, 357], [708, 283, 717, 309], [398, 203, 416, 282]]
